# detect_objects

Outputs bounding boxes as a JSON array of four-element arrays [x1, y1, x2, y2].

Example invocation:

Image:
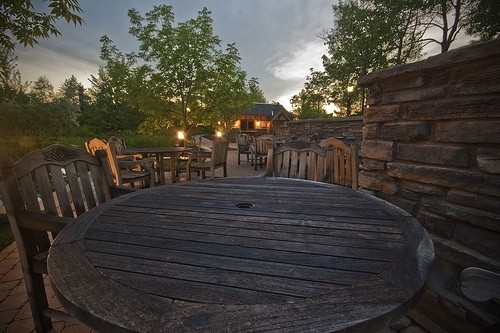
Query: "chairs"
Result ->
[[238, 135, 255, 165], [0, 143, 141, 333], [319, 137, 359, 190], [255, 135, 276, 170], [85, 138, 156, 187], [186, 137, 229, 181], [257, 141, 334, 184]]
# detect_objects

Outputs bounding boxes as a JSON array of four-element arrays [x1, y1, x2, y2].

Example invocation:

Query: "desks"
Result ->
[[122, 147, 194, 184], [47, 177, 435, 333]]
